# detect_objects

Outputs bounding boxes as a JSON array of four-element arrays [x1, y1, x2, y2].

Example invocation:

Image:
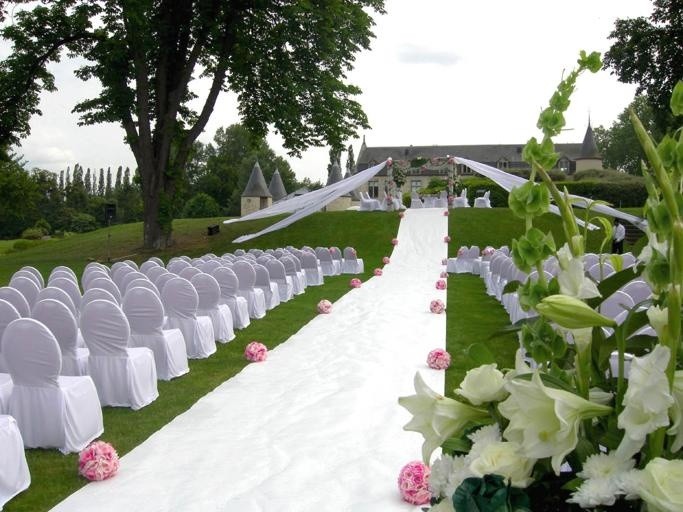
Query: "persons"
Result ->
[[609, 218, 625, 254]]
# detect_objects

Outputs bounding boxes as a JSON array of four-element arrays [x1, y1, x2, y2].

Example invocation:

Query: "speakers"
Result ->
[[208, 224, 219, 235]]
